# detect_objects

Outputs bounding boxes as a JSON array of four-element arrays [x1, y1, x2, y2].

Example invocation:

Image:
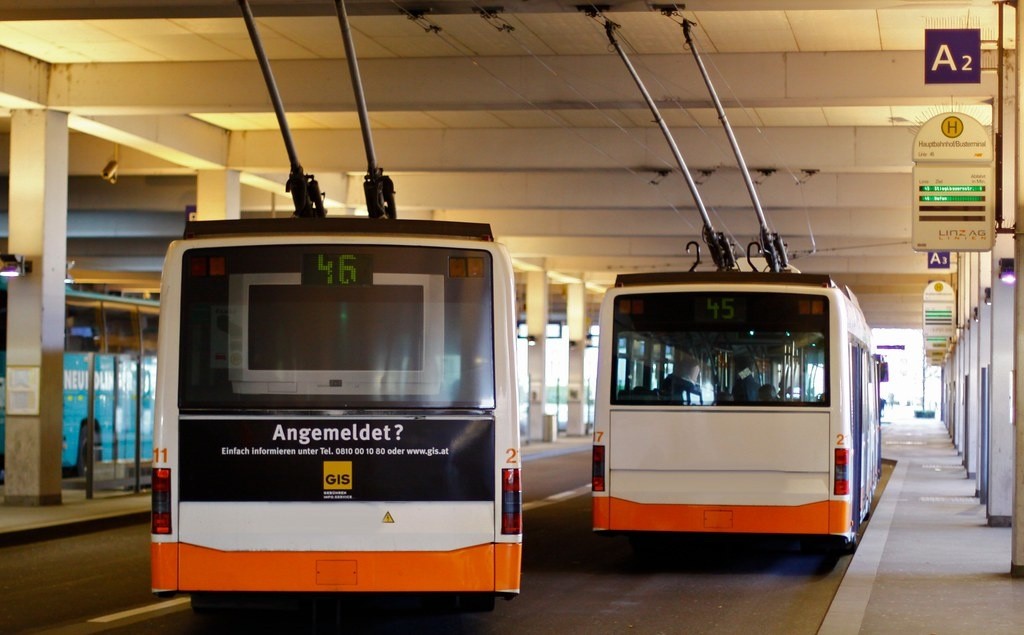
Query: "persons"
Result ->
[[759, 384, 779, 401], [673, 346, 700, 382], [731, 359, 762, 400]]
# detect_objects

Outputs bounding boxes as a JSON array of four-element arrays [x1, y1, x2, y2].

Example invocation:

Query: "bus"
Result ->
[[152, 0, 527, 602], [589, 14, 889, 544], [0, 276, 160, 477]]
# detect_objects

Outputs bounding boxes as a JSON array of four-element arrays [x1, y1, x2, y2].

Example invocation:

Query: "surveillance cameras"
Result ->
[[100, 160, 118, 180]]
